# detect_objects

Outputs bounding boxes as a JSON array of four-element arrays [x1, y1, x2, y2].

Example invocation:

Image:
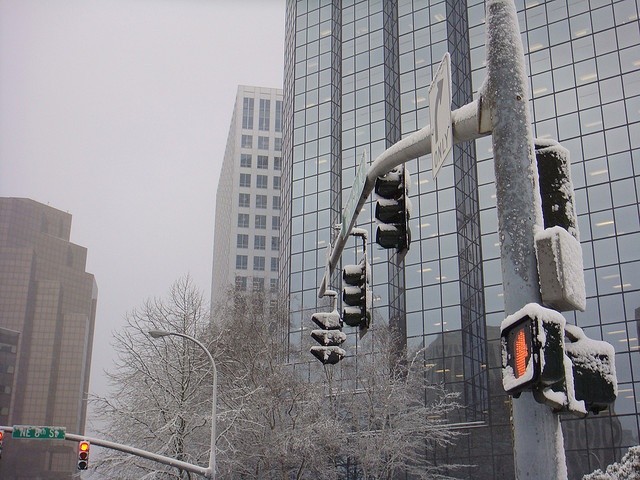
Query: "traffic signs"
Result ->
[[12, 426, 68, 441]]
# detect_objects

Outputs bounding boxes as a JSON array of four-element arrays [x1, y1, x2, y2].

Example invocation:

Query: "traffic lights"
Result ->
[[500, 309, 564, 399], [375, 162, 412, 266], [77, 440, 89, 469], [573, 342, 616, 417], [342, 256, 372, 340], [309, 311, 347, 366]]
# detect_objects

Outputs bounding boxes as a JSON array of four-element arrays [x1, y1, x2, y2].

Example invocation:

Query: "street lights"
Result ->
[[147, 325, 219, 479]]
